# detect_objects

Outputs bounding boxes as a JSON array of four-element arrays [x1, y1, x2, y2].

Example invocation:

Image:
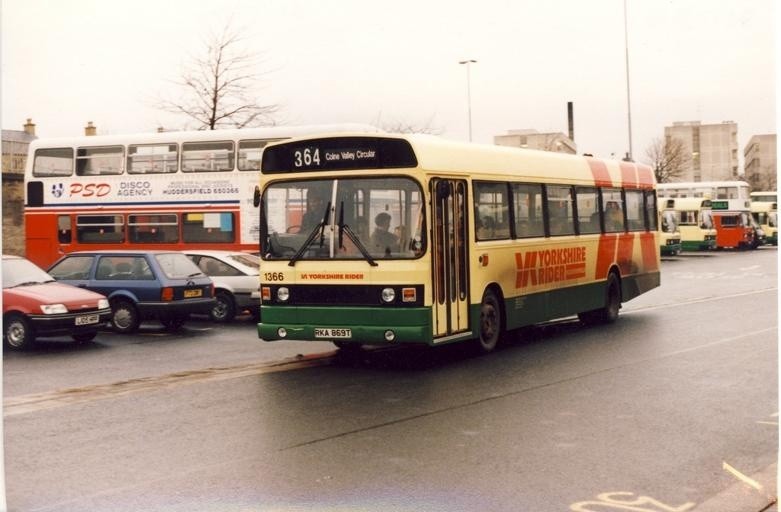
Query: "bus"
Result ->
[[251, 134, 664, 357], [25, 132, 305, 270], [525, 180, 777, 256]]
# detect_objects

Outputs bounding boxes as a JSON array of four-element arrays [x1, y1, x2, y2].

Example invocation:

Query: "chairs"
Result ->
[[516, 211, 640, 237], [59, 225, 233, 242]]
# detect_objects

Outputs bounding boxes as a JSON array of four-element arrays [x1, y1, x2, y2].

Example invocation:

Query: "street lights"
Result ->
[[460, 60, 478, 140]]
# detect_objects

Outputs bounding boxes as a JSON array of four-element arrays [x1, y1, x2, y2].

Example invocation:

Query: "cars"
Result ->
[[44, 250, 219, 335], [181, 249, 263, 322], [2, 254, 113, 352]]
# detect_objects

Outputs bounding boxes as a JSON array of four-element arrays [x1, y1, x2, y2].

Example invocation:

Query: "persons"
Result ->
[[298, 188, 327, 236], [369, 212, 404, 253], [394, 226, 409, 239]]
